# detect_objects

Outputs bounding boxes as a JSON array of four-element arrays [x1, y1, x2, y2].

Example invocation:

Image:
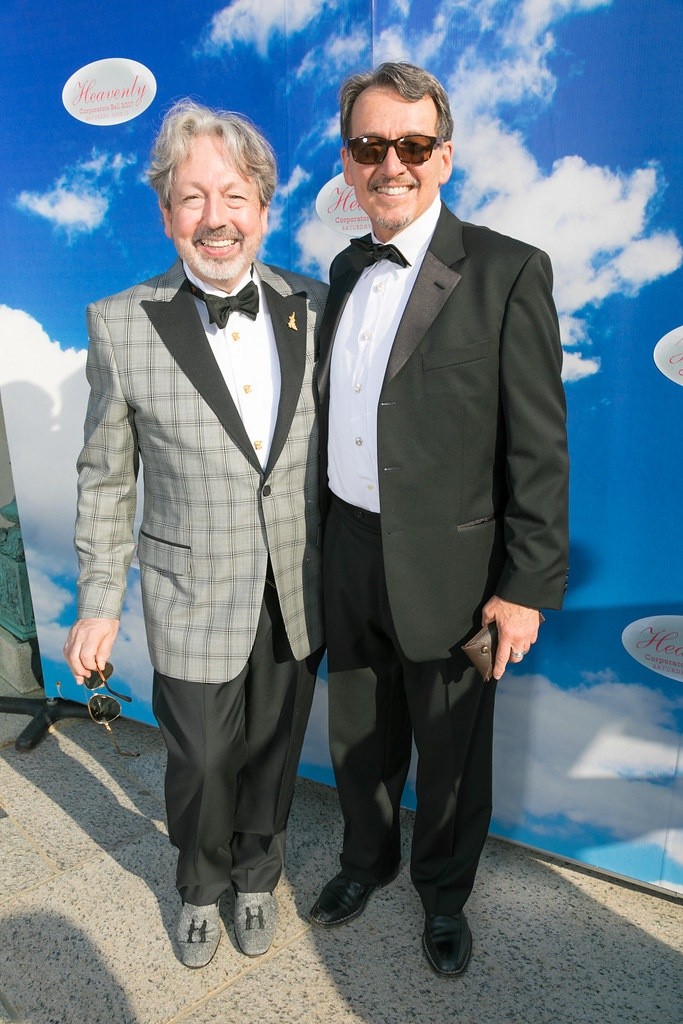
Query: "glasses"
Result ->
[[85, 660, 140, 757], [344, 134, 440, 165]]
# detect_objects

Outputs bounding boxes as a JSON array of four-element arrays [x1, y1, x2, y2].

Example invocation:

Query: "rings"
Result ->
[[511, 650, 525, 658]]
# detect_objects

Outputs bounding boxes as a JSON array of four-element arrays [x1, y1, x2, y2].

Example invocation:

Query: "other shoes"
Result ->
[[233, 891, 278, 958], [177, 899, 220, 968]]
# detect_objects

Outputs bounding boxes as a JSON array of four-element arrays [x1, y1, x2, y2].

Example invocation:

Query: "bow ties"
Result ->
[[204, 280, 259, 329], [350, 237, 407, 269]]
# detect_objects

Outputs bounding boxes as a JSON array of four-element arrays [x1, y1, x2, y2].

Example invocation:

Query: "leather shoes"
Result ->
[[311, 864, 399, 927], [422, 910, 473, 980]]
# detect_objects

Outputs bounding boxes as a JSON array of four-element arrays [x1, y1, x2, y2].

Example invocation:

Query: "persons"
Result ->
[[308, 62, 571, 979], [66, 100, 332, 967]]
